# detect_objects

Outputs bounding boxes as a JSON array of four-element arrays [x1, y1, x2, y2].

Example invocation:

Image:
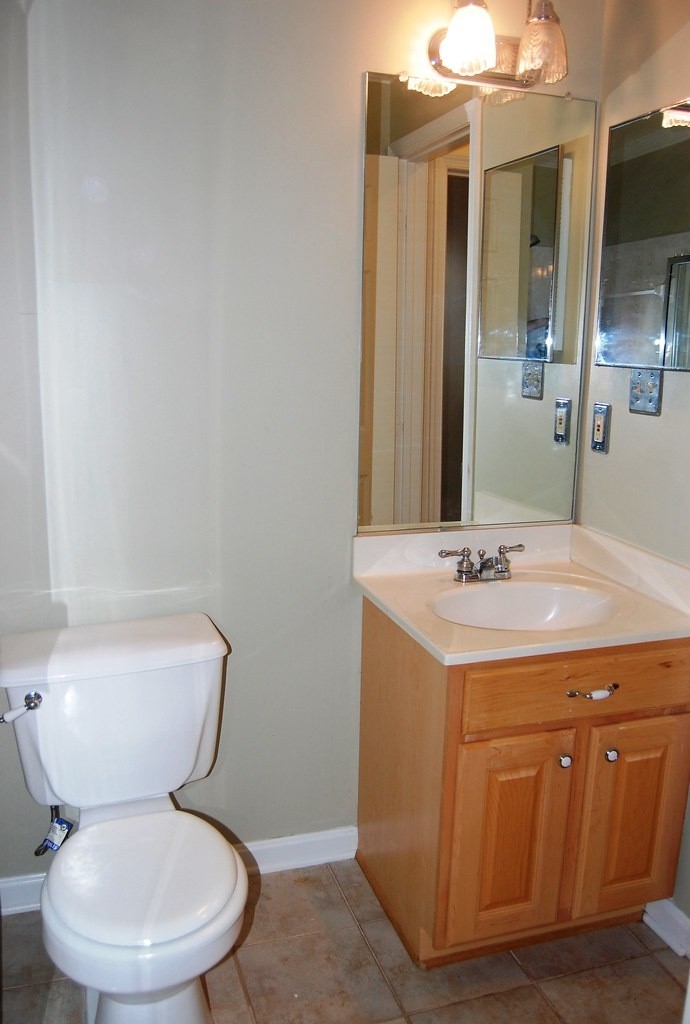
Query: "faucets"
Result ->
[[437, 541, 527, 579]]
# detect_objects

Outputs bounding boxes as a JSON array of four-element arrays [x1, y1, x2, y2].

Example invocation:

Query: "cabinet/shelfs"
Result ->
[[353, 594, 689, 972]]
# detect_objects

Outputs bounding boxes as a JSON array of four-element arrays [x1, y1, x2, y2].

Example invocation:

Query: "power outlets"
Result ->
[[554, 397, 572, 446], [590, 403, 611, 454]]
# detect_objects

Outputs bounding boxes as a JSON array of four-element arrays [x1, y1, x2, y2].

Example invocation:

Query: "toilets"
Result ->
[[1, 613, 246, 1024]]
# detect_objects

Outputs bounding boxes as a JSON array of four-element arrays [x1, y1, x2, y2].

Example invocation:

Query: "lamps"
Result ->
[[399, 16, 525, 105], [427, 0, 569, 90], [661, 103, 690, 128]]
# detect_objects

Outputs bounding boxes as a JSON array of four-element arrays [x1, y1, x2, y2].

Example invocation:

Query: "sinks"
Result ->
[[428, 580, 621, 632]]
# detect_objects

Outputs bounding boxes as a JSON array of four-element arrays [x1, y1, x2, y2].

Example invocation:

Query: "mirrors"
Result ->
[[593, 97, 690, 372], [658, 254, 689, 368], [474, 144, 564, 363], [356, 65, 599, 538]]
